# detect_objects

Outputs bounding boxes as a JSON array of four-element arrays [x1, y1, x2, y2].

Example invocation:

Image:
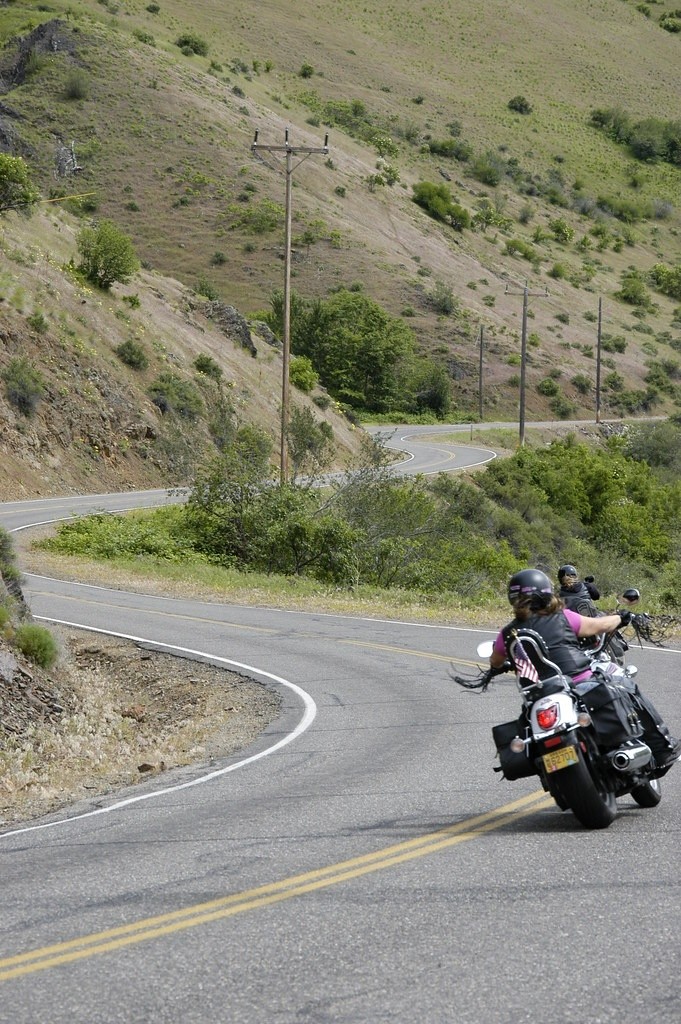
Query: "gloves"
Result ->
[[614, 609, 633, 629]]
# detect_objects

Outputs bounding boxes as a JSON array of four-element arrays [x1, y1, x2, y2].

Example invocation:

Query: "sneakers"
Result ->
[[654, 745, 681, 766]]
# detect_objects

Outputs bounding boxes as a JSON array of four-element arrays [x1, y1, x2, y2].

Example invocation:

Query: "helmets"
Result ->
[[557, 564, 578, 585], [507, 568, 551, 607]]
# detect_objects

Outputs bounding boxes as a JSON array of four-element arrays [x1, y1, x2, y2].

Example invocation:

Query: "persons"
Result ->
[[554, 565, 628, 651], [489, 566, 681, 763]]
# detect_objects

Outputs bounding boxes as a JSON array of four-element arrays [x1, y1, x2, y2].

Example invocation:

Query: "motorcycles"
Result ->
[[555, 577, 629, 664], [478, 590, 681, 828]]
